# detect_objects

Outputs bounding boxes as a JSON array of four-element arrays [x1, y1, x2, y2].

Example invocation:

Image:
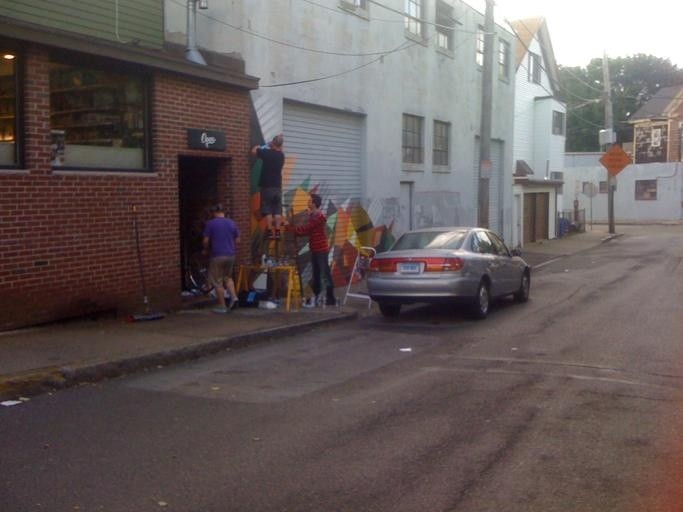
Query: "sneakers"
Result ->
[[212, 298, 238, 313], [267, 232, 280, 239]]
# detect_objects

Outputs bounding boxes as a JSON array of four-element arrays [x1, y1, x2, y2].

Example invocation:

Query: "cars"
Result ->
[[366, 227, 530, 317]]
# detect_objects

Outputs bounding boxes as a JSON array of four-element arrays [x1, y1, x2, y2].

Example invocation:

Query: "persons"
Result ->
[[282, 194, 338, 308], [251, 133, 285, 239], [201, 204, 241, 313]]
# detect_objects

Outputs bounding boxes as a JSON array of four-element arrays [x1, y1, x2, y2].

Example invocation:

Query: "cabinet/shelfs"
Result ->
[[0, 82, 122, 146]]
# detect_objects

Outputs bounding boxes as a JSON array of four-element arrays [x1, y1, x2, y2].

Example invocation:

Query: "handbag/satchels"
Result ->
[[238, 291, 267, 307]]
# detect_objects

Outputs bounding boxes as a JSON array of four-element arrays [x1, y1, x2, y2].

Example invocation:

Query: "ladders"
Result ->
[[259, 204, 306, 307]]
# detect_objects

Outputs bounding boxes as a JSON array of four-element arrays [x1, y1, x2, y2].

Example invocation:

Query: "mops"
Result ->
[[129, 205, 168, 322]]
